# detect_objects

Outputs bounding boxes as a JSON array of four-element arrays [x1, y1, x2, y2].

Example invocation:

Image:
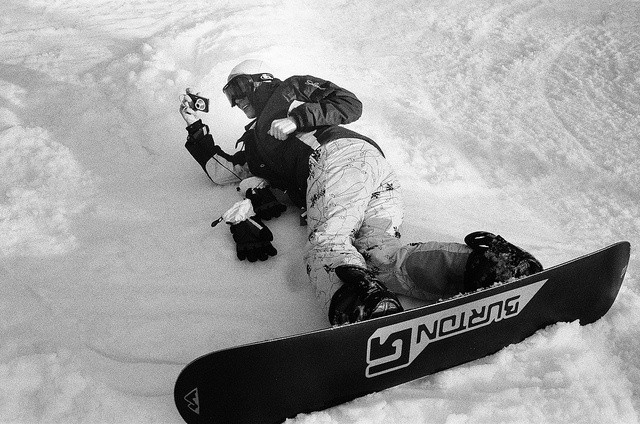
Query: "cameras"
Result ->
[[187, 92, 209, 113]]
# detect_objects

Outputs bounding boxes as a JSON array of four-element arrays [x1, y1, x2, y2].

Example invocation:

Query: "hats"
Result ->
[[227, 58, 275, 81]]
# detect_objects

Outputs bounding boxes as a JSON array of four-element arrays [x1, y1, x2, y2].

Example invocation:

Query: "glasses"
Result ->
[[222, 72, 274, 107]]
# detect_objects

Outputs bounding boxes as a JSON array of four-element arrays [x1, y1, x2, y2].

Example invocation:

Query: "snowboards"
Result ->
[[174, 241, 631, 424]]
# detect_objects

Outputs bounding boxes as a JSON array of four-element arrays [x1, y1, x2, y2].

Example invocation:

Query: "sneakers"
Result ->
[[328, 275, 401, 327], [463, 249, 542, 291]]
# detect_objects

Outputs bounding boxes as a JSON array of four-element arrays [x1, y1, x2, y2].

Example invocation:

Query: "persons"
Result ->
[[180, 58, 542, 327]]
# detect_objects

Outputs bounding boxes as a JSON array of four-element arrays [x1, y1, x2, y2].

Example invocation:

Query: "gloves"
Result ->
[[211, 197, 277, 262], [234, 176, 288, 220]]
